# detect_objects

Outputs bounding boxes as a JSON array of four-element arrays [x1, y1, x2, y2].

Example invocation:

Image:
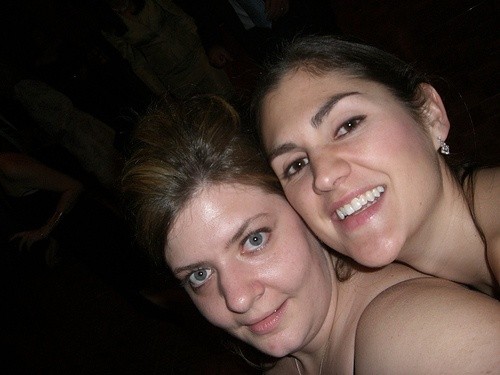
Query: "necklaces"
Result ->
[[292, 345, 329, 375]]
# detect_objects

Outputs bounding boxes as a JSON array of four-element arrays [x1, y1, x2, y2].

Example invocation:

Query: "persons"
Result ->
[[240, 26, 500, 300], [125, 96, 498, 375]]
